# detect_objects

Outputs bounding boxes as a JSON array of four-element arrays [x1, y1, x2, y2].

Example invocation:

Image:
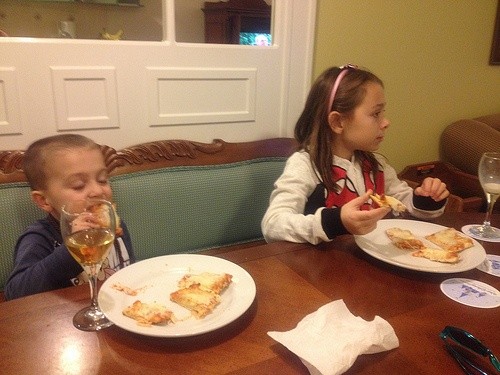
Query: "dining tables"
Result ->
[[0, 210, 500, 375]]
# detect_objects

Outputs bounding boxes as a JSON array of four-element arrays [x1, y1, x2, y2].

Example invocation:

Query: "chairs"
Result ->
[[439, 113, 500, 177]]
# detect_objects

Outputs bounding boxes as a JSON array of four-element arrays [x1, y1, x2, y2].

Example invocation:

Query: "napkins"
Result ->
[[266, 299, 400, 375]]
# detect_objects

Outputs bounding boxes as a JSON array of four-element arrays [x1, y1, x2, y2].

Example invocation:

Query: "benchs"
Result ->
[[0, 137, 303, 304]]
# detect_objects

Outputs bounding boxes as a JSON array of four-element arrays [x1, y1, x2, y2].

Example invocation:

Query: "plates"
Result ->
[[97, 253, 257, 338], [354, 218, 486, 273]]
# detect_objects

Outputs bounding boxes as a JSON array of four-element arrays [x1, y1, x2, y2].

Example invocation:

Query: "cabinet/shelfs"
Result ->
[[201, 0, 272, 44]]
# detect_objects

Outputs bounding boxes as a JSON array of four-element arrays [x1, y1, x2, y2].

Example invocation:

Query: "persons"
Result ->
[[5, 134, 136, 300], [261, 64, 449, 245]]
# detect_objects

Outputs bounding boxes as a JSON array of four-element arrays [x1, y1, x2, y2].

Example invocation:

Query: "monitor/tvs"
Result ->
[[238, 31, 271, 47]]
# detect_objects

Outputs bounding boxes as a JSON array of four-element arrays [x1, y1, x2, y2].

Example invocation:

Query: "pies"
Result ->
[[122, 271, 232, 324], [385, 227, 473, 262], [369, 193, 406, 213], [86, 202, 124, 237]]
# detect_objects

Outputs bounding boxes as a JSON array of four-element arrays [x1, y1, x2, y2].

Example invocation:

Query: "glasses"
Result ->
[[440, 326, 500, 375]]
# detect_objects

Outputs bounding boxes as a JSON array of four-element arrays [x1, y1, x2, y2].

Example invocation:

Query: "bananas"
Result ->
[[98, 28, 123, 40]]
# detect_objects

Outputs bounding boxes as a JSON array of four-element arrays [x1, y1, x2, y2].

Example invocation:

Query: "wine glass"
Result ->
[[470, 152, 500, 239], [59, 199, 116, 331]]
